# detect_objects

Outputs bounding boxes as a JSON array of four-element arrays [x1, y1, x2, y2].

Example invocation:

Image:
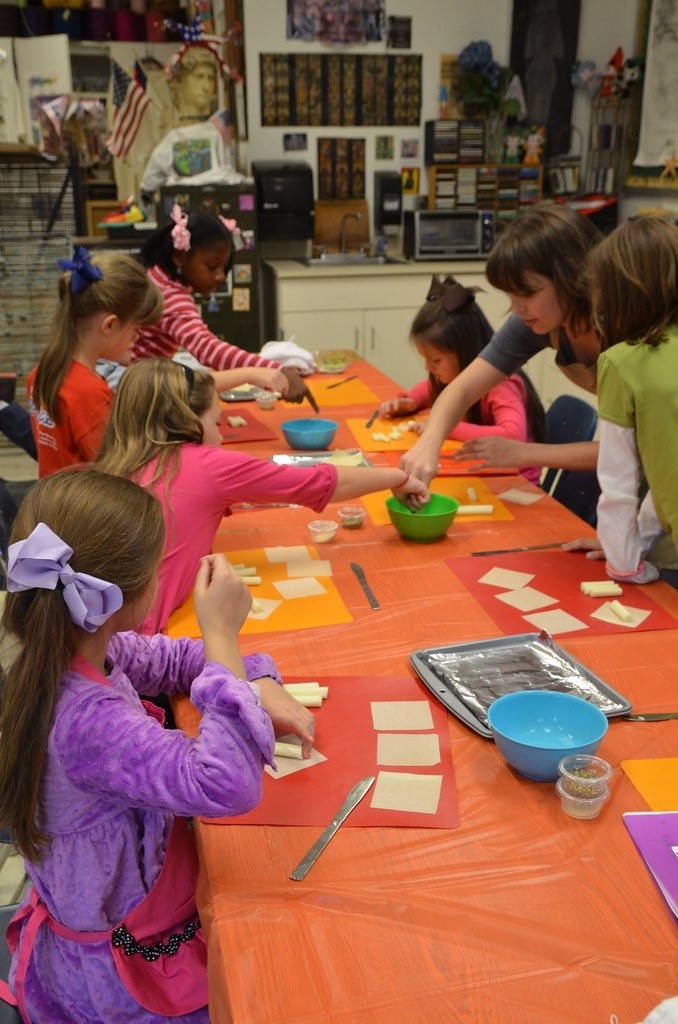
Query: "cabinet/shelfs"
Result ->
[[275, 275, 540, 399], [64, 40, 116, 184]]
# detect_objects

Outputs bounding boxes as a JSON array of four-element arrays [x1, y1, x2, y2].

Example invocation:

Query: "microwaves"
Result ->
[[403, 208, 497, 262]]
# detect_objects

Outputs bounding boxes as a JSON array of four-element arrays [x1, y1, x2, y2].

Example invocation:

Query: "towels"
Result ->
[[259, 341, 318, 375]]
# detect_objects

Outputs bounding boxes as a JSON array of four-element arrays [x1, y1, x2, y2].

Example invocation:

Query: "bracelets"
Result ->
[[391, 472, 409, 489]]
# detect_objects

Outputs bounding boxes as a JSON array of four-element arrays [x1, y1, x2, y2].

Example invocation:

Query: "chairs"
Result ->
[[537, 396, 599, 496]]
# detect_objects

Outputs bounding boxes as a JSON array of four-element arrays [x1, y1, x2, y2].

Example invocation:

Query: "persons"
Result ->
[[89, 206, 320, 412], [378, 275, 548, 493], [398, 196, 648, 528], [0, 466, 321, 1024], [90, 356, 432, 639], [26, 245, 288, 478], [561, 213, 678, 585]]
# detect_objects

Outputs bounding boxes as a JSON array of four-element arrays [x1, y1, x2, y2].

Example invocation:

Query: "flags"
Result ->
[[103, 58, 151, 164], [210, 106, 234, 145]]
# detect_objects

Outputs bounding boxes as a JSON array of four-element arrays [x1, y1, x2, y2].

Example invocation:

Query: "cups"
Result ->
[[0, 373, 17, 403], [88, 9, 111, 41], [144, 11, 170, 41], [110, 10, 138, 41], [51, 6, 82, 35], [0, 4, 49, 37]]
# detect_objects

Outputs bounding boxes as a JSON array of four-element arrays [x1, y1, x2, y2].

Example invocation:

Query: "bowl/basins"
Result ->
[[307, 519, 339, 544], [487, 690, 609, 781], [314, 361, 350, 373], [557, 754, 613, 798], [555, 777, 610, 819], [280, 418, 339, 450], [254, 392, 278, 410], [385, 491, 460, 543], [338, 505, 367, 529]]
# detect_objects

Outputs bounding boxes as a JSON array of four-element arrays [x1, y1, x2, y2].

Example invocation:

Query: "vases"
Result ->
[[483, 121, 506, 163]]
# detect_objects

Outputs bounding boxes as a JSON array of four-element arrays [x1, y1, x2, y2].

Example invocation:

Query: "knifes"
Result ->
[[623, 713, 678, 722], [351, 562, 381, 610], [470, 543, 561, 556], [222, 434, 240, 437], [365, 410, 379, 428], [326, 375, 357, 389], [289, 776, 376, 881]]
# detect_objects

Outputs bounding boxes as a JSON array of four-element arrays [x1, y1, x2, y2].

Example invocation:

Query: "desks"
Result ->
[[165, 351, 678, 1024]]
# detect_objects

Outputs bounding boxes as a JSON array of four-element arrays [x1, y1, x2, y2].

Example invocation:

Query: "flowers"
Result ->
[[456, 40, 523, 149]]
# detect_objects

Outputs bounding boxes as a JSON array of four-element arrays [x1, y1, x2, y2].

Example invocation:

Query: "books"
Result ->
[[430, 118, 483, 158], [430, 166, 541, 212]]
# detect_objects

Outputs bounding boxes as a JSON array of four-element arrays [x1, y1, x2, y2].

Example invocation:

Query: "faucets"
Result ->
[[310, 253, 408, 265], [340, 212, 363, 252]]
